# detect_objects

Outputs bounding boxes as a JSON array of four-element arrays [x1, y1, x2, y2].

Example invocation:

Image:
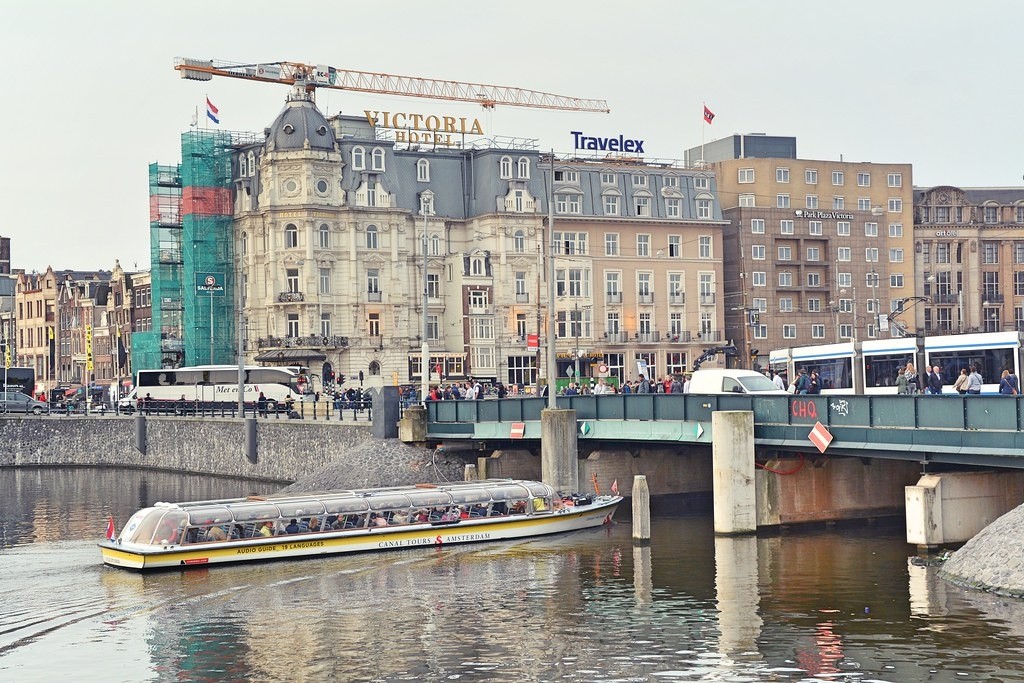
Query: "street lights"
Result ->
[[739, 273, 751, 369]]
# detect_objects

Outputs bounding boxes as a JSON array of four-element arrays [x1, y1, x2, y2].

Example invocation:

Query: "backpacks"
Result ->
[[658, 384, 663, 393], [802, 375, 813, 392]]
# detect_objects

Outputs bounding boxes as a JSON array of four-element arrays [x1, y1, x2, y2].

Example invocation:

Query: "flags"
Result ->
[[104, 515, 116, 543], [206, 96, 220, 124], [704, 105, 714, 124]]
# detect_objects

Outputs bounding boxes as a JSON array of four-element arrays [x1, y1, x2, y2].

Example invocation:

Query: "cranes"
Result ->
[[175, 55, 613, 113]]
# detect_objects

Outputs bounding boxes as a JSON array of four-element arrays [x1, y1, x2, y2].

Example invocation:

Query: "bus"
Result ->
[[136, 366, 308, 411], [766, 331, 1023, 393]]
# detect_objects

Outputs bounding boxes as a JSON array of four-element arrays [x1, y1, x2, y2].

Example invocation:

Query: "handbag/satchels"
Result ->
[[643, 380, 654, 393], [1012, 388, 1017, 395], [956, 386, 960, 392]]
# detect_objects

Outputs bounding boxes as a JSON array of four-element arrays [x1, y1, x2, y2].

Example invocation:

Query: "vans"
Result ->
[[119, 387, 136, 414], [689, 369, 792, 396]]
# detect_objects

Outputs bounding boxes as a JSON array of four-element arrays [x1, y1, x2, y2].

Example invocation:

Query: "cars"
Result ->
[[0, 392, 48, 415]]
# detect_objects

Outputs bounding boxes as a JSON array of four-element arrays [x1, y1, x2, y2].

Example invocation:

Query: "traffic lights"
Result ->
[[750, 349, 758, 355], [337, 373, 345, 384], [331, 372, 335, 378]]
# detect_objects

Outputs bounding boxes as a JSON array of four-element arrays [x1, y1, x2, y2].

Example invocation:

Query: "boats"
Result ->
[[95, 478, 625, 570]]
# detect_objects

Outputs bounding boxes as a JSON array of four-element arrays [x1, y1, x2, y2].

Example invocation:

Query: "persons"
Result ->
[[772, 369, 820, 395], [39, 392, 47, 402], [153, 489, 545, 543], [60, 395, 68, 408], [561, 373, 691, 394], [257, 387, 363, 417], [180, 395, 189, 416], [398, 380, 549, 407], [895, 362, 1020, 395], [144, 394, 151, 415]]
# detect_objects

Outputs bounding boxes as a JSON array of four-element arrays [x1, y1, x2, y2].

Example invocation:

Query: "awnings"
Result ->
[[65, 389, 78, 396]]
